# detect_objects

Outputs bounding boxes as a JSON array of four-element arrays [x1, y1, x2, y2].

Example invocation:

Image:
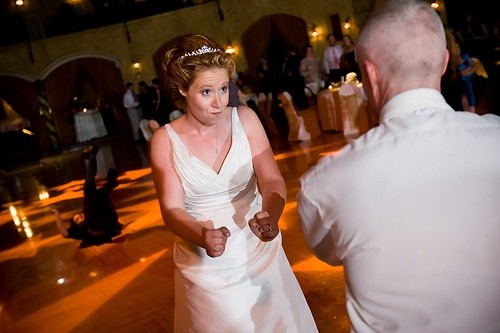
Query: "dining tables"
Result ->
[[72, 109, 108, 143], [317, 83, 366, 131]]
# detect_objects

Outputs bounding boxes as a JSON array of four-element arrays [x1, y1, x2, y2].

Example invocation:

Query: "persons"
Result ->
[[149, 33, 319, 333], [49, 144, 119, 241], [122, 78, 164, 141], [236, 32, 362, 110], [66, 91, 83, 136], [96, 99, 108, 122], [440, 27, 476, 113], [295, 0, 500, 333]]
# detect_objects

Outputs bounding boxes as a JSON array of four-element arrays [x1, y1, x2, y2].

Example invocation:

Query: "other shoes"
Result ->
[[81, 146, 98, 158], [108, 168, 118, 186]]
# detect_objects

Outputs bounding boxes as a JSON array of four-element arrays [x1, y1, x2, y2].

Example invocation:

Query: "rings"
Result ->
[[269, 223, 275, 231], [214, 245, 215, 252]]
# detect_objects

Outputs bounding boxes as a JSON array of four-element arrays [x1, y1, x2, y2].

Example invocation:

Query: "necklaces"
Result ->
[[194, 122, 219, 153]]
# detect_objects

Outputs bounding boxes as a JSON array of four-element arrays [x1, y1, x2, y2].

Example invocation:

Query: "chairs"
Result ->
[[140, 111, 183, 147], [235, 80, 370, 146]]
